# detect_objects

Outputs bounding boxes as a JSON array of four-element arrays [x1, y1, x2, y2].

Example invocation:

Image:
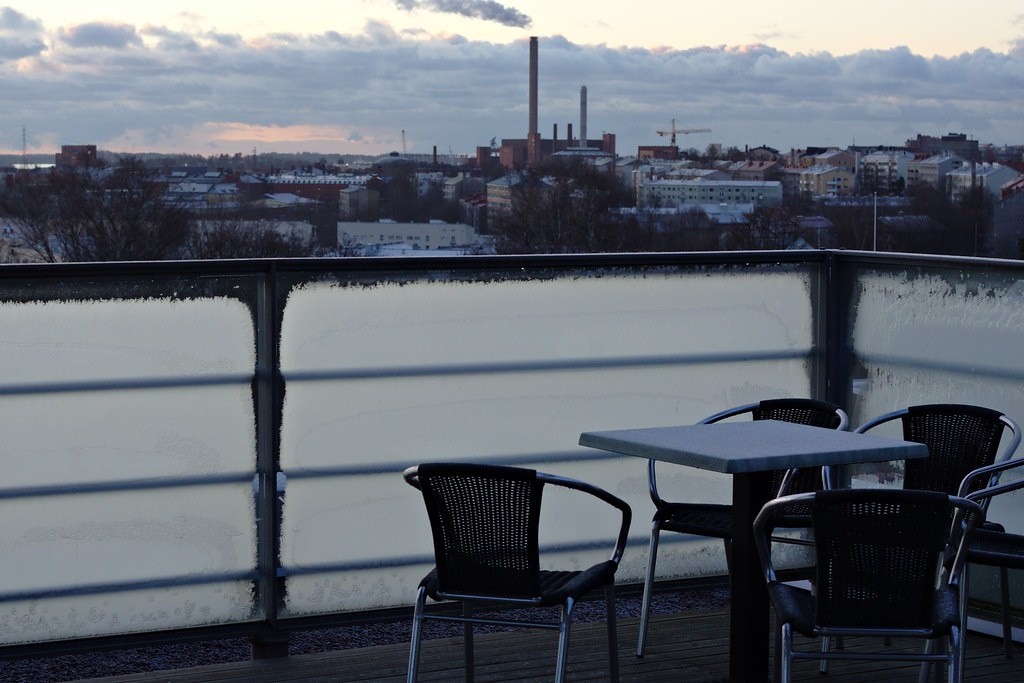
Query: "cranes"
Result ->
[[655, 118, 713, 147]]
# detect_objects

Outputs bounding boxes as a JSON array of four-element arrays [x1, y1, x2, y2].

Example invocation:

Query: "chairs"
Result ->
[[931, 456, 1024, 683], [818, 404, 1022, 673], [752, 486, 984, 683], [402, 461, 632, 683], [636, 396, 847, 671]]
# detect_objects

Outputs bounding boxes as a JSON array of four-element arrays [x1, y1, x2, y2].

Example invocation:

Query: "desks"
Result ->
[[579, 417, 929, 683]]
